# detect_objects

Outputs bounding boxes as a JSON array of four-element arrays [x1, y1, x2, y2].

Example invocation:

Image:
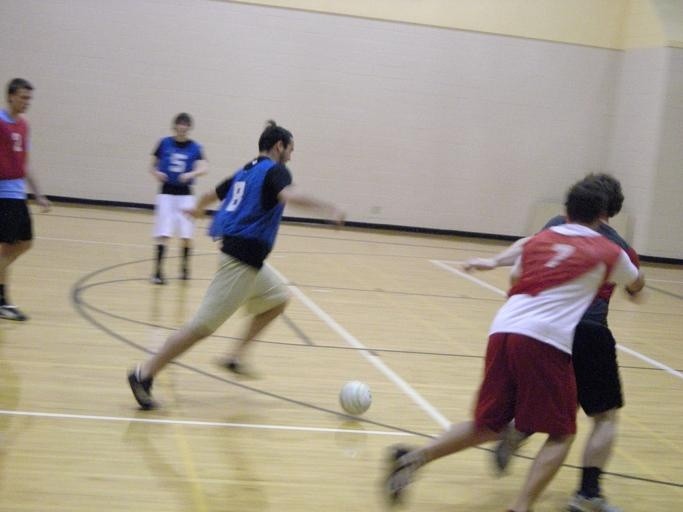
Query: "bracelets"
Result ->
[[623, 285, 644, 296]]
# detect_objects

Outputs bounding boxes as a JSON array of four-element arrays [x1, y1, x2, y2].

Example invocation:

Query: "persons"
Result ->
[[147, 111, 211, 284], [496, 172, 642, 510], [377, 180, 646, 511], [0, 75, 51, 326], [123, 120, 346, 409]]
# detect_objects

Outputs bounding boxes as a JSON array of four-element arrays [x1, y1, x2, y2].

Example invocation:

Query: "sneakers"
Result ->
[[0, 303, 30, 321], [127, 366, 158, 410], [384, 443, 414, 505], [569, 490, 621, 512]]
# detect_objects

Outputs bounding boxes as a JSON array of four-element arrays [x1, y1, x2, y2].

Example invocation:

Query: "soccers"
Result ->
[[338, 379, 371, 413]]
